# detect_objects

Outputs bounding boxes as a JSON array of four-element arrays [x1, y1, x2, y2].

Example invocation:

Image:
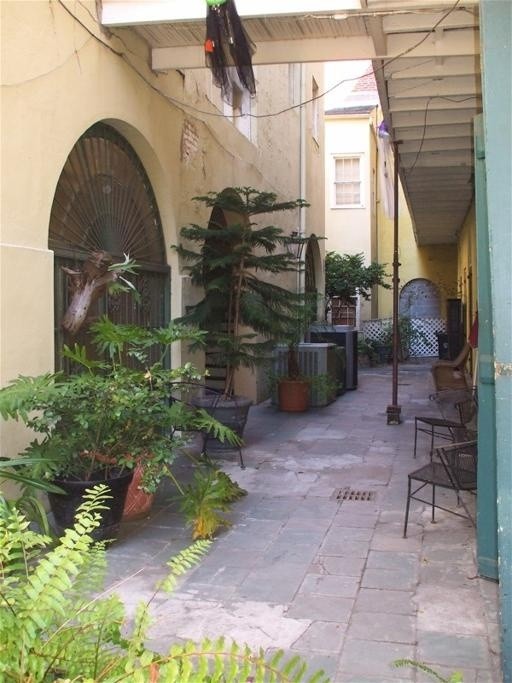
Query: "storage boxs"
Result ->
[[303, 324, 358, 389], [270, 341, 337, 407]]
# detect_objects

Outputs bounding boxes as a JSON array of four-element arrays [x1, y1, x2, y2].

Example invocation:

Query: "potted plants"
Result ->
[[0, 312, 249, 540], [255, 291, 343, 411], [168, 184, 329, 450]]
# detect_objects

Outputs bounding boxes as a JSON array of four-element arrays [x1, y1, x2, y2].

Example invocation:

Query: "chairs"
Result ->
[[413, 385, 478, 462], [164, 380, 248, 468], [402, 439, 477, 538]]
